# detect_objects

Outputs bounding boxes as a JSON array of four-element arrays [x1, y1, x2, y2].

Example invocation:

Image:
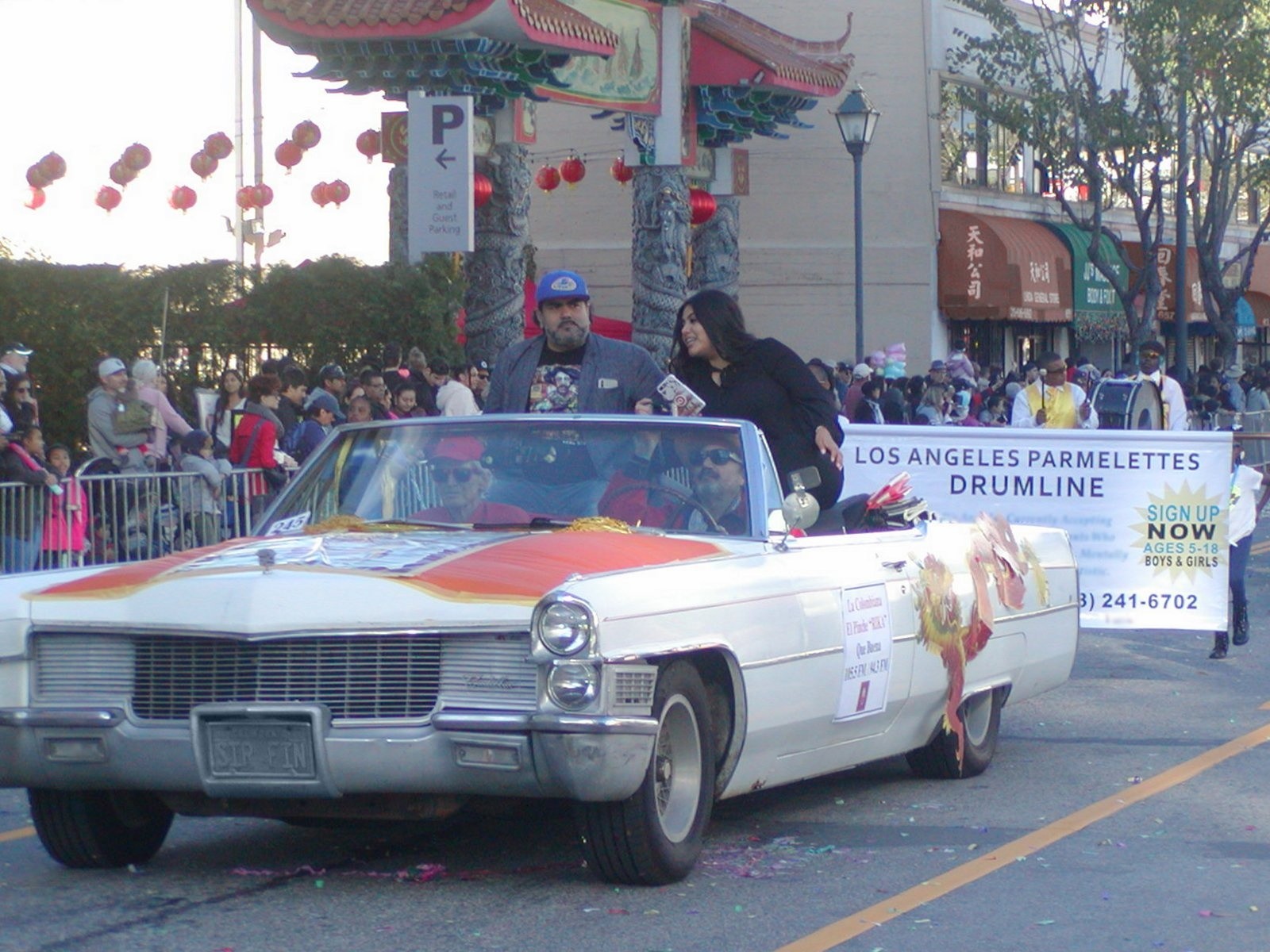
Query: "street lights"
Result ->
[[827, 80, 882, 365]]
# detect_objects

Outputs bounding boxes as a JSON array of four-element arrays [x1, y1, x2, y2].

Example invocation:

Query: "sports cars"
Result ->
[[1, 411, 1083, 888]]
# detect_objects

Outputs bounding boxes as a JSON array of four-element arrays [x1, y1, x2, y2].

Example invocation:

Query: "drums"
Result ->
[[1081, 376, 1167, 433]]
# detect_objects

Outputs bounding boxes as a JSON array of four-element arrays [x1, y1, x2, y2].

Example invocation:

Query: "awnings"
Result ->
[[1118, 240, 1223, 325], [1034, 219, 1133, 343], [936, 207, 1074, 325], [1161, 286, 1257, 340]]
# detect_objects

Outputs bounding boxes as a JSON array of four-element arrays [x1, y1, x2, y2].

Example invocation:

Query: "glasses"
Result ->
[[688, 449, 744, 466], [432, 467, 482, 485], [478, 374, 491, 381], [1140, 353, 1160, 359], [15, 388, 31, 394], [367, 384, 387, 388], [1046, 364, 1068, 374]]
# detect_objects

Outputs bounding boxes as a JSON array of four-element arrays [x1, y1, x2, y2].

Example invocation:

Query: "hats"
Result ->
[[472, 359, 493, 372], [98, 357, 128, 379], [1140, 341, 1165, 355], [1224, 365, 1246, 377], [929, 360, 950, 372], [89, 510, 112, 530], [853, 363, 874, 379], [427, 436, 486, 468], [536, 270, 590, 303], [184, 429, 209, 451], [674, 430, 741, 468], [311, 395, 347, 419], [836, 362, 853, 371], [321, 366, 345, 379], [4, 341, 33, 355]]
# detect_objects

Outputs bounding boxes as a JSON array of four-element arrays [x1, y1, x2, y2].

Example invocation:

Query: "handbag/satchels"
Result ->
[[222, 464, 249, 497]]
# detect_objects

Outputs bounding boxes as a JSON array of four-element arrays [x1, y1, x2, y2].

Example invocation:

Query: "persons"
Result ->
[[1, 340, 494, 575], [480, 267, 665, 518], [596, 398, 806, 541], [1206, 424, 1270, 659], [799, 341, 1270, 474], [665, 289, 846, 513], [405, 431, 534, 526]]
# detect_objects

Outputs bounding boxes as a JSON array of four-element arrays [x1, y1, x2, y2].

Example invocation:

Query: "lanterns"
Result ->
[[290, 119, 320, 150], [357, 128, 382, 162], [685, 180, 718, 277], [610, 155, 633, 188], [534, 164, 560, 194], [23, 132, 350, 210], [560, 153, 586, 190], [474, 169, 493, 211]]
